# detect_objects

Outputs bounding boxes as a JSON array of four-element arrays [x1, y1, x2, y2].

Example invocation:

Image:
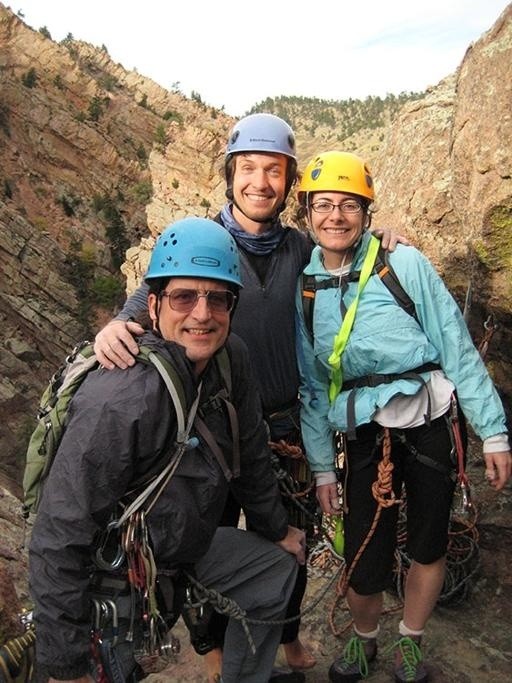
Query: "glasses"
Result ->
[[310, 198, 362, 216], [159, 284, 235, 316]]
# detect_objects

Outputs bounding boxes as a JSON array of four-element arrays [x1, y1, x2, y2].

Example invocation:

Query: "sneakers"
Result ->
[[328, 629, 378, 681], [391, 628, 427, 683]]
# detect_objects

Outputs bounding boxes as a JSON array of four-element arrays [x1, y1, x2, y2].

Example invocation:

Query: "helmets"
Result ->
[[143, 214, 245, 293], [297, 147, 379, 208], [223, 111, 298, 164]]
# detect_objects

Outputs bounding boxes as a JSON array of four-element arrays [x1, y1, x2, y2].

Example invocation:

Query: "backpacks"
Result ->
[[15, 332, 242, 518]]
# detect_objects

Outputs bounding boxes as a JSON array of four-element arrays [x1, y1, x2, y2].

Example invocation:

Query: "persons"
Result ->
[[91, 107, 411, 682], [23, 214, 311, 682], [288, 146, 512, 683]]
